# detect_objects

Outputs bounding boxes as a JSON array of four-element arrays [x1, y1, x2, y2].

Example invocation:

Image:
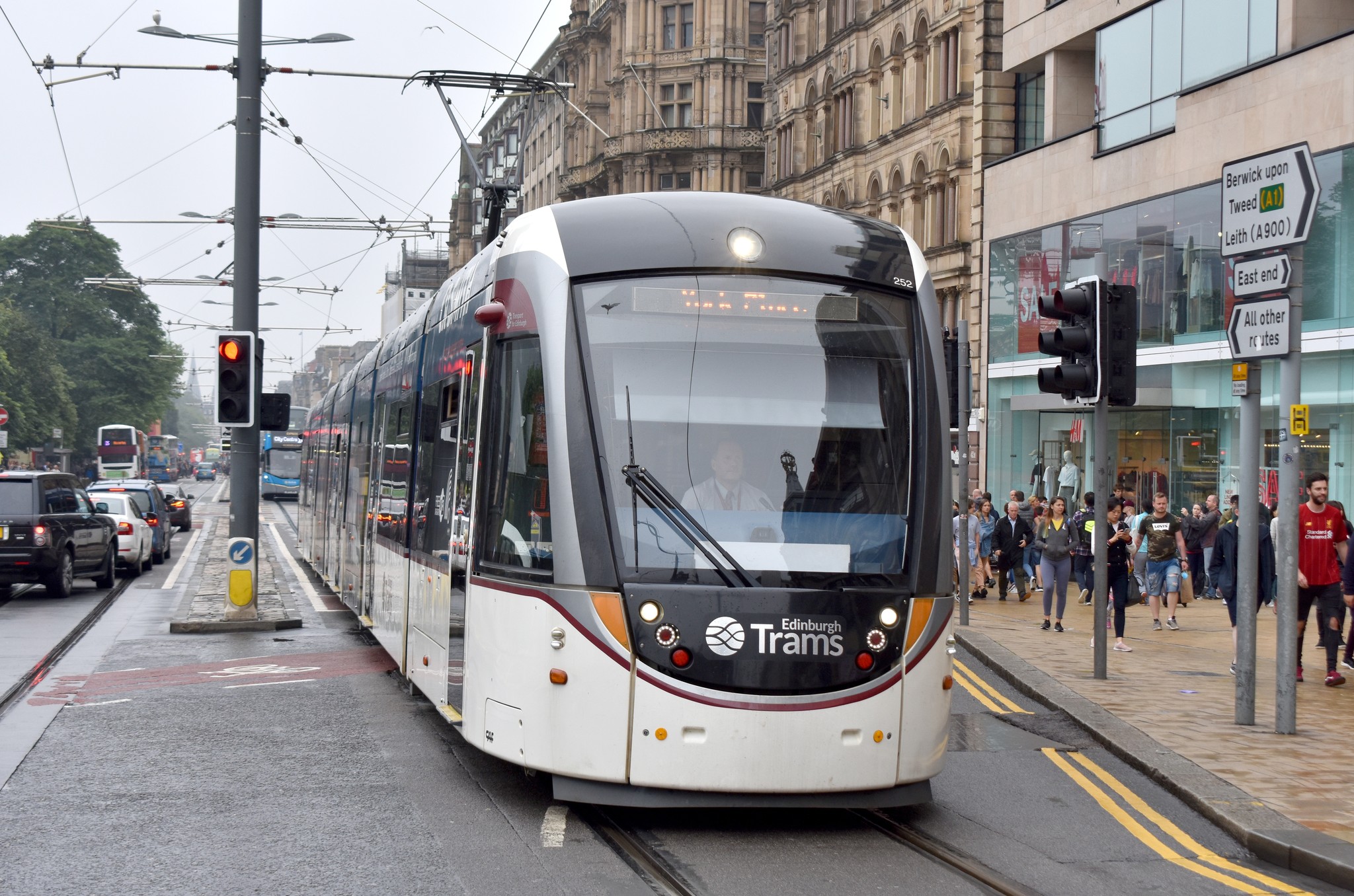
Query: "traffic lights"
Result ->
[[1038, 275, 1107, 405], [215, 331, 255, 427]]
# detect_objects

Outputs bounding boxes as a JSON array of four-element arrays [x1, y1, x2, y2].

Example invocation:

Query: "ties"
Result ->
[[725, 492, 734, 511]]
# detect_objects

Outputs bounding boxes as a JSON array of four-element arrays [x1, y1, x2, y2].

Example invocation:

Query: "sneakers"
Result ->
[[1053, 621, 1064, 632], [1315, 637, 1326, 649], [1296, 666, 1303, 682], [1040, 619, 1051, 631], [1338, 635, 1347, 648], [1091, 637, 1095, 647], [1113, 642, 1132, 652], [1166, 615, 1179, 630], [1325, 670, 1345, 687], [1153, 619, 1162, 630], [1340, 653, 1354, 670], [1230, 660, 1236, 675]]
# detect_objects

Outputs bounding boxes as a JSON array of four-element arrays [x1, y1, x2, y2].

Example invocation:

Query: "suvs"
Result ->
[[84, 479, 175, 564], [0, 470, 119, 599]]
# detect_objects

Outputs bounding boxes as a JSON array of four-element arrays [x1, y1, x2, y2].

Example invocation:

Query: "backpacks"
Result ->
[[1079, 509, 1094, 549]]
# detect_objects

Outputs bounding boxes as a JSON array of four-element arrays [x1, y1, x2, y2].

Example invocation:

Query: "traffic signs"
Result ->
[[1226, 295, 1291, 362], [1233, 250, 1293, 299], [1220, 141, 1322, 260]]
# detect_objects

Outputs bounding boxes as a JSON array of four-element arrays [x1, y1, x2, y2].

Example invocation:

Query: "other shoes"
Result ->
[[1273, 600, 1277, 615], [1195, 593, 1227, 605], [1142, 603, 1150, 606], [954, 577, 996, 603], [999, 576, 1044, 601], [1106, 619, 1111, 629], [1078, 589, 1092, 605], [1139, 591, 1146, 604]]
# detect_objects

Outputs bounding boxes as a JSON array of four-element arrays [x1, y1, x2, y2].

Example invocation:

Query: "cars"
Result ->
[[136, 484, 195, 532], [431, 506, 469, 569], [75, 492, 158, 578], [194, 462, 217, 481]]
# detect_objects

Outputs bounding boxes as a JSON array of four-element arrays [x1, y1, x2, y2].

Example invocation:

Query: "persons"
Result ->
[[951, 488, 1000, 604], [990, 501, 1034, 601], [1057, 450, 1080, 518], [1296, 472, 1349, 686], [71, 460, 98, 488], [1308, 500, 1354, 670], [678, 440, 794, 586], [1029, 449, 1046, 496], [1035, 496, 1080, 632], [1069, 492, 1097, 606], [1091, 498, 1133, 652], [1207, 504, 1276, 676], [1110, 482, 1239, 608], [996, 489, 1051, 593], [0, 460, 61, 472], [1127, 492, 1189, 631]]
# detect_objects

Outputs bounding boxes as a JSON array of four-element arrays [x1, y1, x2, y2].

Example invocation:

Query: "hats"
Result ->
[[1123, 499, 1134, 508], [1028, 450, 1043, 456]]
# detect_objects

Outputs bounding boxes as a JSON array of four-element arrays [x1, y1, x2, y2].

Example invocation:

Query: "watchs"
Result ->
[[1181, 559, 1188, 563]]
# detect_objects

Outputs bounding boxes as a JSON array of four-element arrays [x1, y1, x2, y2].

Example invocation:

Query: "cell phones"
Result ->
[[1123, 528, 1131, 533], [1198, 510, 1203, 518]]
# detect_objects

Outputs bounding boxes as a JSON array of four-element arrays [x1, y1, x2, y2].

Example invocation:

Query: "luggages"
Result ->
[[1161, 576, 1187, 607]]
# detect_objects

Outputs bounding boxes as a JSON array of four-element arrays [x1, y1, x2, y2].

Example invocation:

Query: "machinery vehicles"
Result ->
[[260, 406, 311, 500]]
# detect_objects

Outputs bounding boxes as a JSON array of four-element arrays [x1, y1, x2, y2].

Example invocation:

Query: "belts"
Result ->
[[1060, 486, 1071, 489]]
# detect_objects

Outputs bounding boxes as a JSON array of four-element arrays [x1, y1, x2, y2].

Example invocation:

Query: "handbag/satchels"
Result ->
[[1180, 570, 1194, 603], [1125, 574, 1141, 608]]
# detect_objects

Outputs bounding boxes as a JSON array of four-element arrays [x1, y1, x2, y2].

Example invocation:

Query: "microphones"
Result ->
[[1000, 552, 1005, 555]]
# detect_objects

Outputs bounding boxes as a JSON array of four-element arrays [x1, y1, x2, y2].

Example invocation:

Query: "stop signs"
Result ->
[[0, 408, 8, 425]]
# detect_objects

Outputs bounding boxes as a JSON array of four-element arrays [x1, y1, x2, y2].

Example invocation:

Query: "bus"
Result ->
[[309, 444, 423, 523], [146, 434, 179, 483], [298, 191, 956, 808], [205, 448, 220, 463], [97, 424, 148, 480]]
